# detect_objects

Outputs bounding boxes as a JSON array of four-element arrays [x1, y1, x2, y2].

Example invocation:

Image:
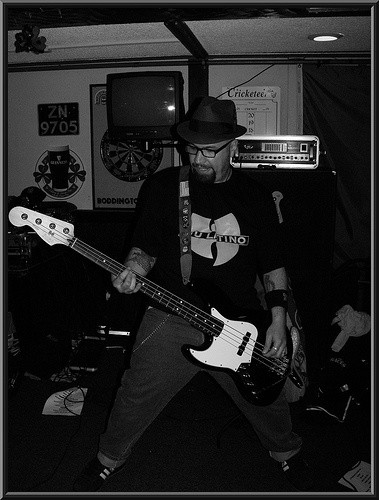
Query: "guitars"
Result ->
[[10, 196, 294, 406]]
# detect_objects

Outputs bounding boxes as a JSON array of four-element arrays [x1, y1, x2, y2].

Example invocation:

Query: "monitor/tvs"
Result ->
[[106, 71, 184, 139]]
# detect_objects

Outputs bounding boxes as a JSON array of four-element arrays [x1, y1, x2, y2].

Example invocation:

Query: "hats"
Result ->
[[178, 96, 247, 143]]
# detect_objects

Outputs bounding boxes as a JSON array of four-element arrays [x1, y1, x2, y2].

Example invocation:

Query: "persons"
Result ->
[[73, 97, 328, 492]]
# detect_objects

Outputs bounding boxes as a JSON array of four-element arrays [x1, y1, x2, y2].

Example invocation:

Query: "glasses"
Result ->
[[184, 138, 235, 158]]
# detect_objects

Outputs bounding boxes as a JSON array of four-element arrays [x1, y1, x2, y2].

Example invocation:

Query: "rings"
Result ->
[[273, 345, 278, 352]]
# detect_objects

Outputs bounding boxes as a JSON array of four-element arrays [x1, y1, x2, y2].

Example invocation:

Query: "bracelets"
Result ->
[[265, 289, 288, 310]]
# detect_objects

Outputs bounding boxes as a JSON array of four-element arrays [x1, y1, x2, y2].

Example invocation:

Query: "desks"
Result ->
[[8, 253, 77, 383]]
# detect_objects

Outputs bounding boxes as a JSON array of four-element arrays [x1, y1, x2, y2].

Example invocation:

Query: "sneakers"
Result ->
[[72, 456, 128, 492], [279, 452, 315, 492]]
